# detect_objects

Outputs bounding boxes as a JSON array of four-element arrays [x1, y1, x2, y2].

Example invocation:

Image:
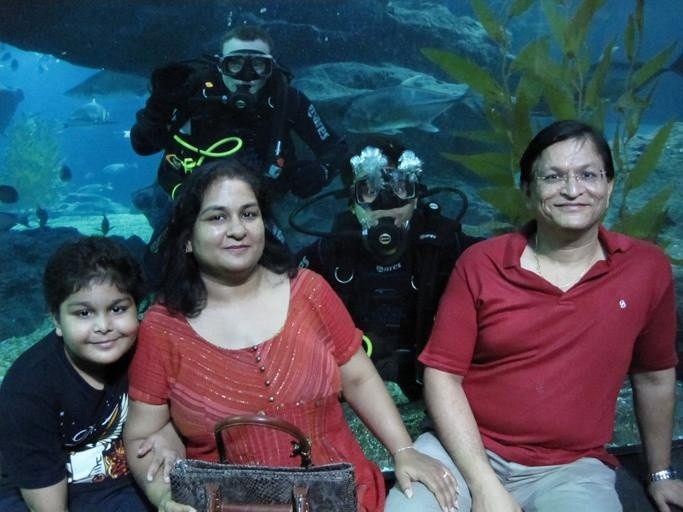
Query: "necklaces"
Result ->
[[532, 232, 600, 288]]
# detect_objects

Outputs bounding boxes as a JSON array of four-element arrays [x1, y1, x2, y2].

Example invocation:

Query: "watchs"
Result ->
[[645, 464, 678, 483]]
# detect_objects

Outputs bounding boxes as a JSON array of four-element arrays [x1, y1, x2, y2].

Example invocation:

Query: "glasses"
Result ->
[[219, 49, 276, 81], [354, 167, 417, 211], [535, 168, 606, 183]]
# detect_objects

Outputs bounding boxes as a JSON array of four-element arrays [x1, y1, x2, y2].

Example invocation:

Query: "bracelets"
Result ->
[[390, 445, 414, 457]]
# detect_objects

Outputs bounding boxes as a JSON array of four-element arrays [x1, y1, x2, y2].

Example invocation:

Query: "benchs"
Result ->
[[380, 441, 683, 511]]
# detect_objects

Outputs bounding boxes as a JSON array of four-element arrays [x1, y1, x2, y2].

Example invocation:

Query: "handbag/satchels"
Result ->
[[169, 416, 357, 512]]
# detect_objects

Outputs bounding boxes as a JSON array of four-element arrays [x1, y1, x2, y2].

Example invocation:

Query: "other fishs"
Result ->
[[0, 48, 146, 239], [337, 81, 480, 139]]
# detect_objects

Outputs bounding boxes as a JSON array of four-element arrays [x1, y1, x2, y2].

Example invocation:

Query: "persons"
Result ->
[[293, 138, 488, 402], [380, 117, 683, 512], [128, 22, 348, 287], [119, 156, 463, 512], [0, 237, 188, 512]]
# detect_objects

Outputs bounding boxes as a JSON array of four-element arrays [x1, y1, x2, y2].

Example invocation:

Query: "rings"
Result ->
[[442, 472, 448, 479]]
